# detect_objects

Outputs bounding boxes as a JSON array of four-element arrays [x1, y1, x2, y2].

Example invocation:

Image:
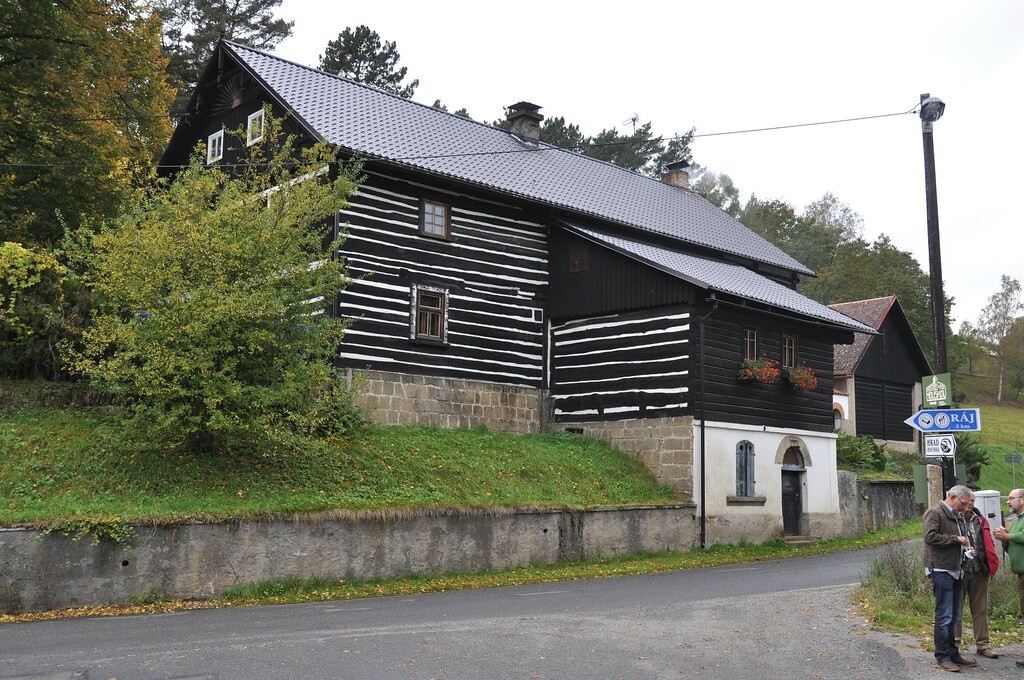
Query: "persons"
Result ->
[[922, 484, 979, 673], [953, 490, 999, 660], [991, 489, 1024, 667]]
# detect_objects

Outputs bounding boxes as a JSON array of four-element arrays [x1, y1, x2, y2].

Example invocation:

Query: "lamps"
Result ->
[[789, 437, 800, 454]]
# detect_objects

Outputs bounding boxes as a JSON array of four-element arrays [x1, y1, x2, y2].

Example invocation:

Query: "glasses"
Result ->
[[956, 496, 968, 507], [1007, 497, 1022, 499]]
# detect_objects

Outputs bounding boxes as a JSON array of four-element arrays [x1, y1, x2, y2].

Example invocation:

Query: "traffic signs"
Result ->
[[903, 407, 982, 433]]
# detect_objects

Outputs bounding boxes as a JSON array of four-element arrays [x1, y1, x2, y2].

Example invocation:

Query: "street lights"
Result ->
[[917, 92, 959, 495]]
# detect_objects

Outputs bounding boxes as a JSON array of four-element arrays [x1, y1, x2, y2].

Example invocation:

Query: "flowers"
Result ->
[[740, 352, 782, 385], [787, 360, 817, 393]]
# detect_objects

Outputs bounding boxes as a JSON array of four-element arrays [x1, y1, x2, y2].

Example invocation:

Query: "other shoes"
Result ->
[[1016, 659, 1024, 667], [935, 661, 960, 672], [952, 657, 977, 667], [977, 649, 998, 658]]
